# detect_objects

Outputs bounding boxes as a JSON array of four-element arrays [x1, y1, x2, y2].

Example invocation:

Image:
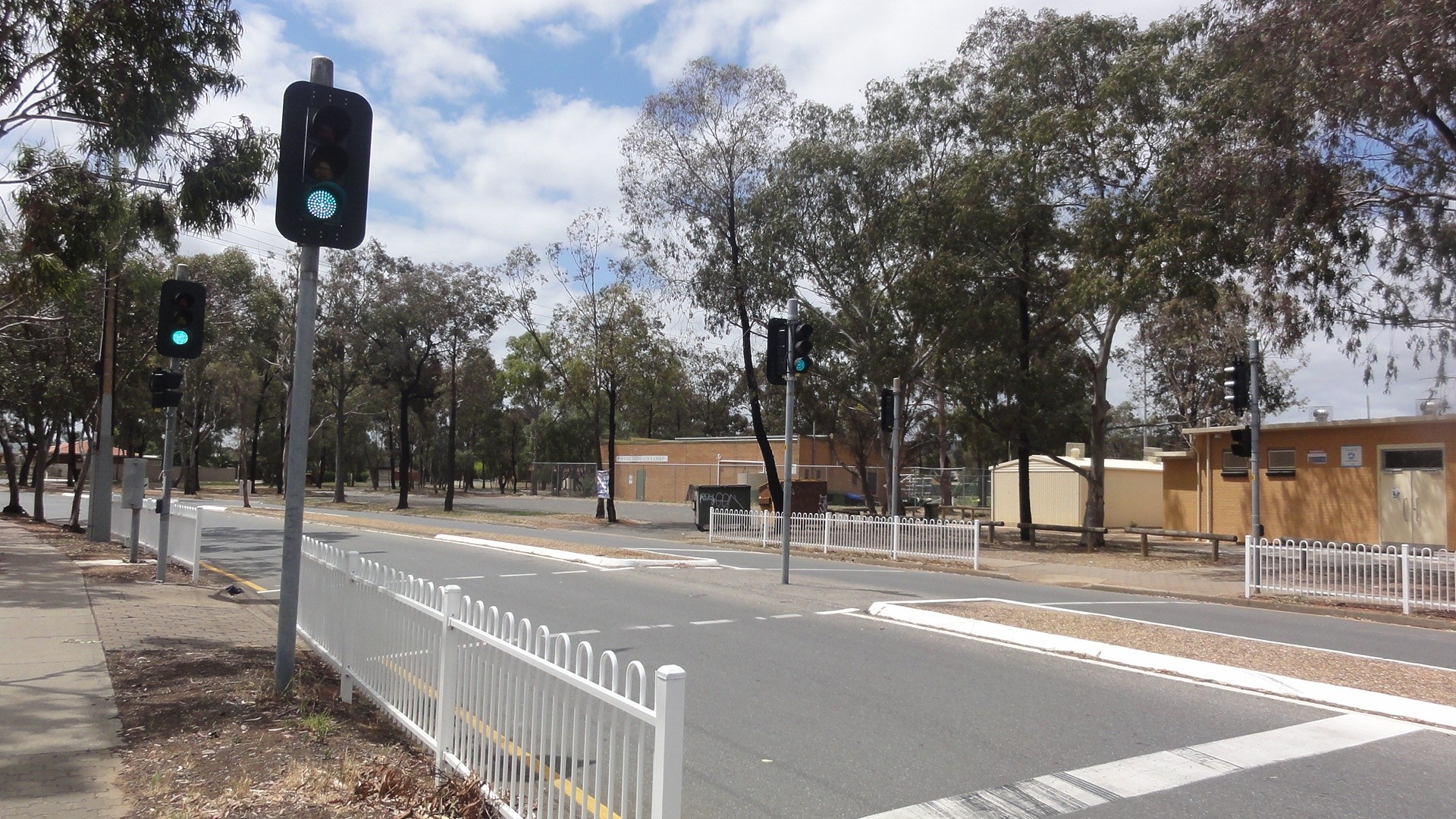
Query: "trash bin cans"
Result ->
[[757, 480, 827, 527], [922, 498, 941, 524], [692, 484, 752, 532]]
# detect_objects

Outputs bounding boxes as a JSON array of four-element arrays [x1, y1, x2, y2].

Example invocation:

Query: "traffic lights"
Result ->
[[157, 279, 209, 358], [148, 367, 185, 408], [1220, 360, 1248, 411], [876, 388, 895, 433], [1230, 426, 1252, 457], [794, 322, 813, 374], [277, 80, 374, 254]]
